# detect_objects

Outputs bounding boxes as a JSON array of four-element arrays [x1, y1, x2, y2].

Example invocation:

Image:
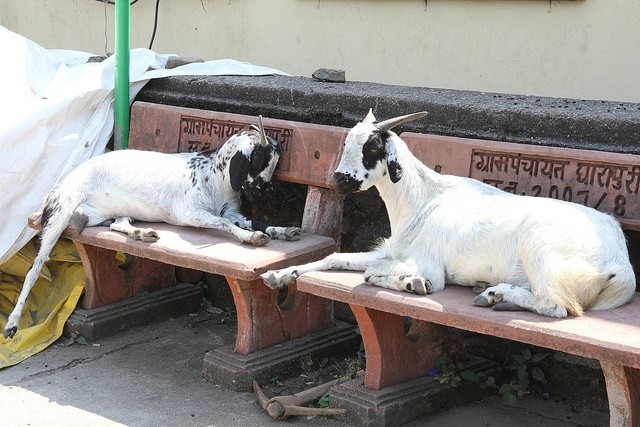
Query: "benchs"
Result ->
[[28, 101, 354, 395], [296, 132, 636, 426]]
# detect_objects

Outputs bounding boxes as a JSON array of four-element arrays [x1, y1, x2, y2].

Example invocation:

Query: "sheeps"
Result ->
[[259, 106, 636, 317], [3, 113, 302, 338]]
